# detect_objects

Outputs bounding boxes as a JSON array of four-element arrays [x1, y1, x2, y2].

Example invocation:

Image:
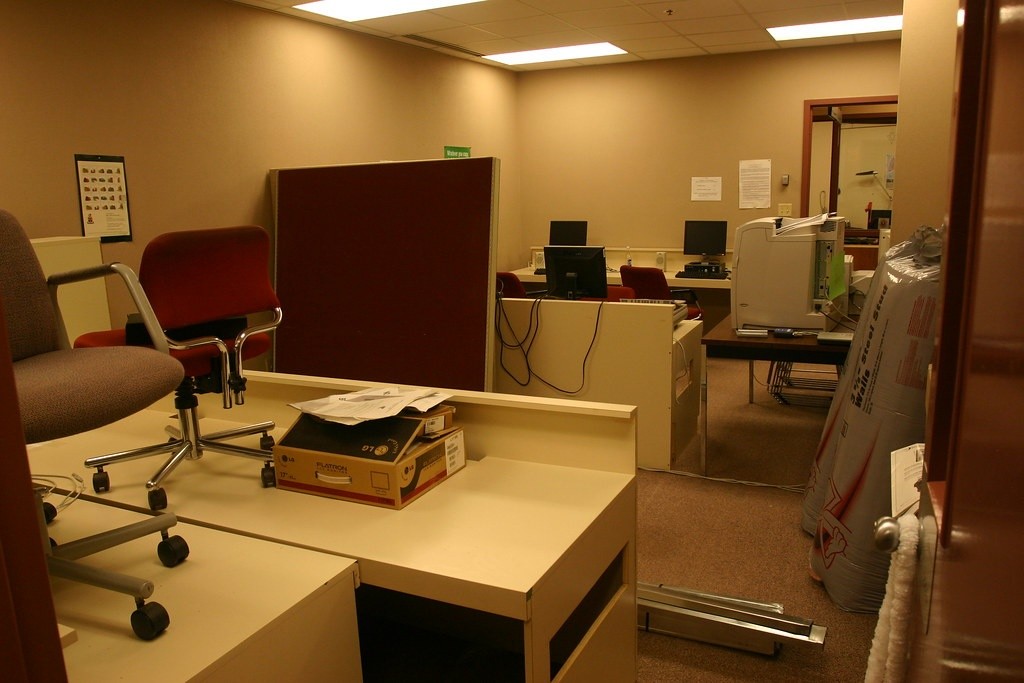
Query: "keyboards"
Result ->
[[675, 271, 728, 279], [534, 268, 546, 275]]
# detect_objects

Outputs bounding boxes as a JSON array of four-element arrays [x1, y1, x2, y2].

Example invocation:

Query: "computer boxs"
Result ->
[[685, 262, 725, 273]]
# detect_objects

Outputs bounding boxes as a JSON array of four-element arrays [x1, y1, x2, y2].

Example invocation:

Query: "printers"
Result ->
[[732, 216, 854, 332]]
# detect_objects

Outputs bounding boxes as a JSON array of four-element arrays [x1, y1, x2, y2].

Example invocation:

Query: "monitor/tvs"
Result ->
[[543, 220, 608, 301], [684, 221, 727, 264]]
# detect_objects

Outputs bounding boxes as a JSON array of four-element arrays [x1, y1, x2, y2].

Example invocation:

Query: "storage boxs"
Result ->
[[272, 403, 466, 511]]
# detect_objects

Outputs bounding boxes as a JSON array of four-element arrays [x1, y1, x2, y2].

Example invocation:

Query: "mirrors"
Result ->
[[798, 95, 898, 250]]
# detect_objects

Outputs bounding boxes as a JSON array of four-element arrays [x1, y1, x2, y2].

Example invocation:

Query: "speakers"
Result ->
[[656, 252, 667, 271], [535, 252, 545, 269]]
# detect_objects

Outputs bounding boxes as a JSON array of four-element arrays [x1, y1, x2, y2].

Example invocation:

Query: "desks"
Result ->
[[36, 489, 365, 683], [26, 370, 639, 683], [701, 314, 858, 477], [495, 296, 702, 471], [510, 247, 734, 288]]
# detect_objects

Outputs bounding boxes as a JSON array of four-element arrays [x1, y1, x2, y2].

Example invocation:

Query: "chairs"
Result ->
[[1, 210, 191, 642], [75, 223, 282, 512], [620, 265, 704, 321], [496, 272, 528, 299], [580, 285, 635, 301]]
